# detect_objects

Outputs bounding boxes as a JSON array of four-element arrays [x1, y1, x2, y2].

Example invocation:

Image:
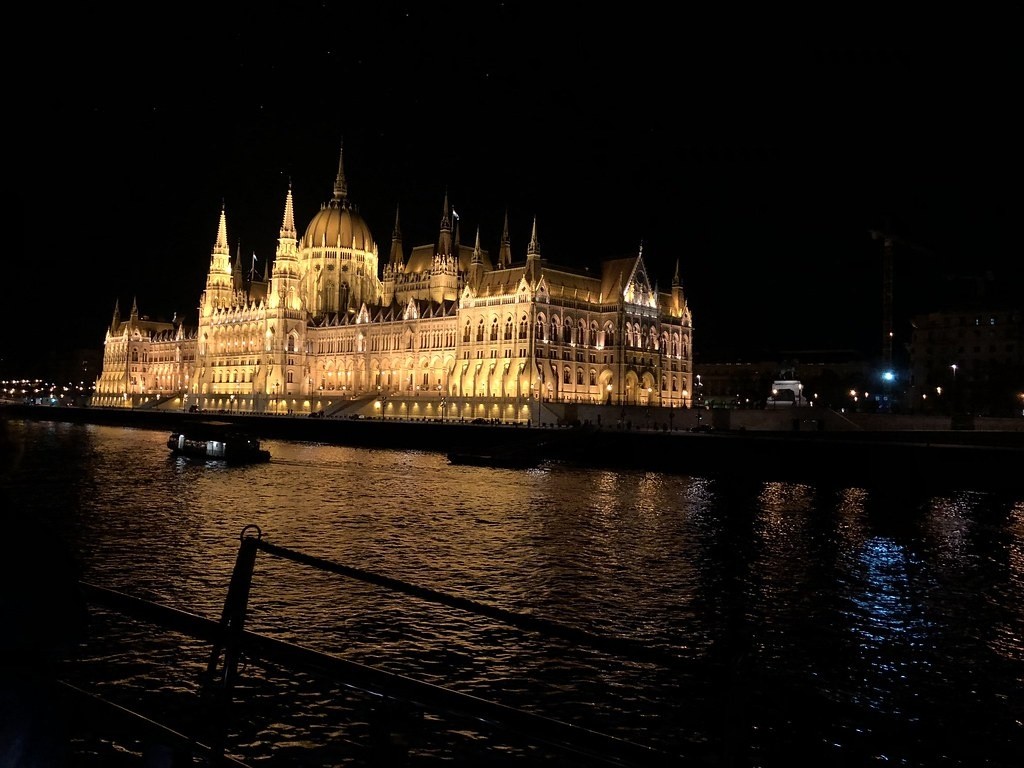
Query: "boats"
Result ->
[[167, 420, 257, 462]]
